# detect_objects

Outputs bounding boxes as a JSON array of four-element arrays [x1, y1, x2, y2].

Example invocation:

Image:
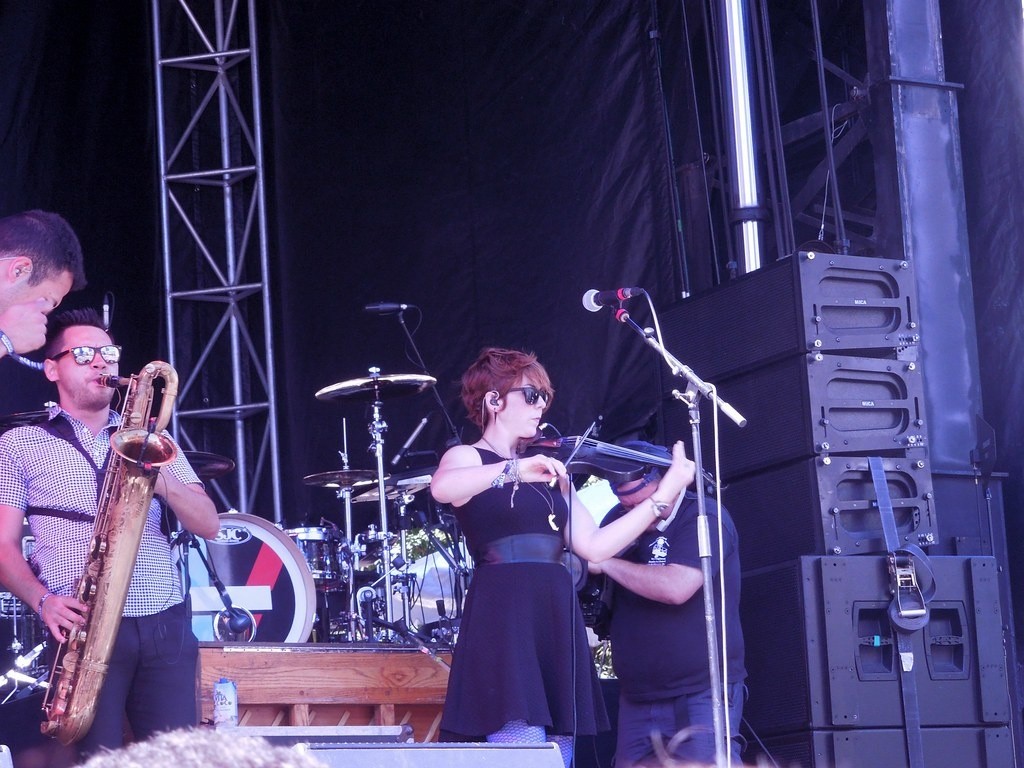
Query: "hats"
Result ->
[[610, 440, 667, 496]]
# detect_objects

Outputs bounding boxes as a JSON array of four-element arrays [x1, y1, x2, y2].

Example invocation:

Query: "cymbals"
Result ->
[[303, 468, 392, 487], [315, 372, 438, 405], [338, 472, 433, 503], [181, 449, 235, 480]]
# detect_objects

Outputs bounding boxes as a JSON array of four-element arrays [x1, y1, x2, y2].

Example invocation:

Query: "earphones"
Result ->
[[16, 269, 21, 276], [491, 398, 499, 406]]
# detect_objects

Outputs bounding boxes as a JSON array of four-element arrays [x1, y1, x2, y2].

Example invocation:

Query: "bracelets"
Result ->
[[493, 459, 519, 508], [649, 495, 669, 516], [0, 331, 45, 370], [38, 592, 54, 623]]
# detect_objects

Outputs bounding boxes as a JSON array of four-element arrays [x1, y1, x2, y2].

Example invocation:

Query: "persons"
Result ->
[[586, 441, 746, 768], [431, 349, 697, 768], [0, 210, 87, 365], [0, 318, 220, 761]]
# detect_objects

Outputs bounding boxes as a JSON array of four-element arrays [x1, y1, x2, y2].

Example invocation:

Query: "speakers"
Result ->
[[303, 741, 566, 768], [646, 248, 1016, 768]]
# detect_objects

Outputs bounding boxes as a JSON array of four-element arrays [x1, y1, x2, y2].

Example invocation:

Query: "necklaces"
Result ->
[[481, 436, 559, 531]]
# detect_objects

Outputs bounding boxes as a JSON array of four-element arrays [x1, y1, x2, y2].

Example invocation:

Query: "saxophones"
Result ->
[[37, 357, 182, 748]]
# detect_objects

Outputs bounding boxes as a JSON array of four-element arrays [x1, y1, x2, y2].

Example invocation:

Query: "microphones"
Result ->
[[103, 294, 109, 329], [583, 287, 645, 312], [365, 302, 416, 316], [215, 579, 251, 633], [392, 411, 432, 465], [148, 419, 157, 433]]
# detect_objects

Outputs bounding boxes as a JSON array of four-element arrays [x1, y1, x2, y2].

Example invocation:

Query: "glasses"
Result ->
[[510, 386, 549, 405], [51, 346, 122, 366]]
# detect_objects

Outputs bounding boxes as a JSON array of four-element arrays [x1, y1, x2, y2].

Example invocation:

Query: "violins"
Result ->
[[525, 434, 732, 495]]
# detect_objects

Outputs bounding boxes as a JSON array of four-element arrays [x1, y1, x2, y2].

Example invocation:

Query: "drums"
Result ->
[[168, 510, 318, 643], [284, 526, 345, 593]]
